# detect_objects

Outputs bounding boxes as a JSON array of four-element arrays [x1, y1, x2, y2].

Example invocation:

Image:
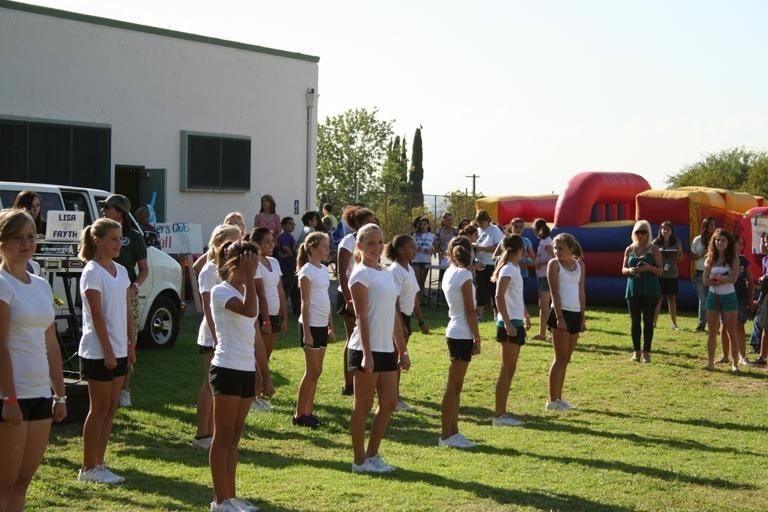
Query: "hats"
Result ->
[[98, 194, 131, 216]]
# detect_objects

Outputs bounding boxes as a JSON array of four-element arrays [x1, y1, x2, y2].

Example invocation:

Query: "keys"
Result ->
[[634, 265, 640, 279]]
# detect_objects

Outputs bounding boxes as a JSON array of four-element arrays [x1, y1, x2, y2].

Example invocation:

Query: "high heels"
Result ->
[[631, 351, 641, 361], [642, 351, 651, 363]]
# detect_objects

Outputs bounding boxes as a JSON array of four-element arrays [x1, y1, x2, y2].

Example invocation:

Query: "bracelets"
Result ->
[[546, 232, 586, 408], [133, 282, 139, 289], [3, 396, 16, 403], [556, 316, 564, 321], [128, 343, 135, 349], [653, 266, 657, 273], [53, 395, 67, 403]]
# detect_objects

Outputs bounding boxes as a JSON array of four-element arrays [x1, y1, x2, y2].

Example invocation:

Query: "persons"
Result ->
[[192, 194, 295, 450], [78, 218, 137, 485], [294, 202, 428, 433], [411, 210, 554, 343], [14, 190, 46, 240], [344, 222, 411, 472], [135, 206, 162, 250], [690, 217, 768, 373], [652, 221, 683, 332], [0, 208, 67, 512], [621, 220, 664, 363], [98, 194, 148, 405], [491, 233, 525, 427], [440, 237, 480, 448], [208, 240, 275, 510]]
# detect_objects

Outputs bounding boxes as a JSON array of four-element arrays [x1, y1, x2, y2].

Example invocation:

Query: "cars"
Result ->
[[0, 177, 186, 351]]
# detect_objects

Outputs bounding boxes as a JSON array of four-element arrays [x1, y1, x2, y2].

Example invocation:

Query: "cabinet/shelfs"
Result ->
[[41, 267, 86, 385]]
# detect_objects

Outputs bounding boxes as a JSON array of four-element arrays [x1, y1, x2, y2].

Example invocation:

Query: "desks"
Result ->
[[424, 263, 448, 306]]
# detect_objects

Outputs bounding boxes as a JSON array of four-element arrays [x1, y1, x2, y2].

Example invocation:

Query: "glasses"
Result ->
[[101, 205, 116, 209], [635, 229, 649, 235]]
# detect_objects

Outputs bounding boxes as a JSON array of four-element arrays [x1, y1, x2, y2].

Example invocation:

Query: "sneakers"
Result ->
[[492, 414, 524, 425], [715, 357, 729, 363], [702, 365, 715, 371], [546, 400, 579, 411], [754, 357, 767, 366], [119, 390, 132, 407], [529, 334, 547, 340], [292, 414, 325, 427], [78, 465, 125, 484], [341, 386, 354, 392], [737, 357, 756, 367], [671, 324, 680, 331], [364, 454, 395, 472], [396, 400, 417, 413], [192, 435, 214, 450], [731, 363, 739, 374], [439, 432, 480, 448], [250, 398, 274, 411], [224, 496, 261, 512], [353, 457, 386, 474], [209, 498, 250, 512], [693, 327, 705, 333]]
